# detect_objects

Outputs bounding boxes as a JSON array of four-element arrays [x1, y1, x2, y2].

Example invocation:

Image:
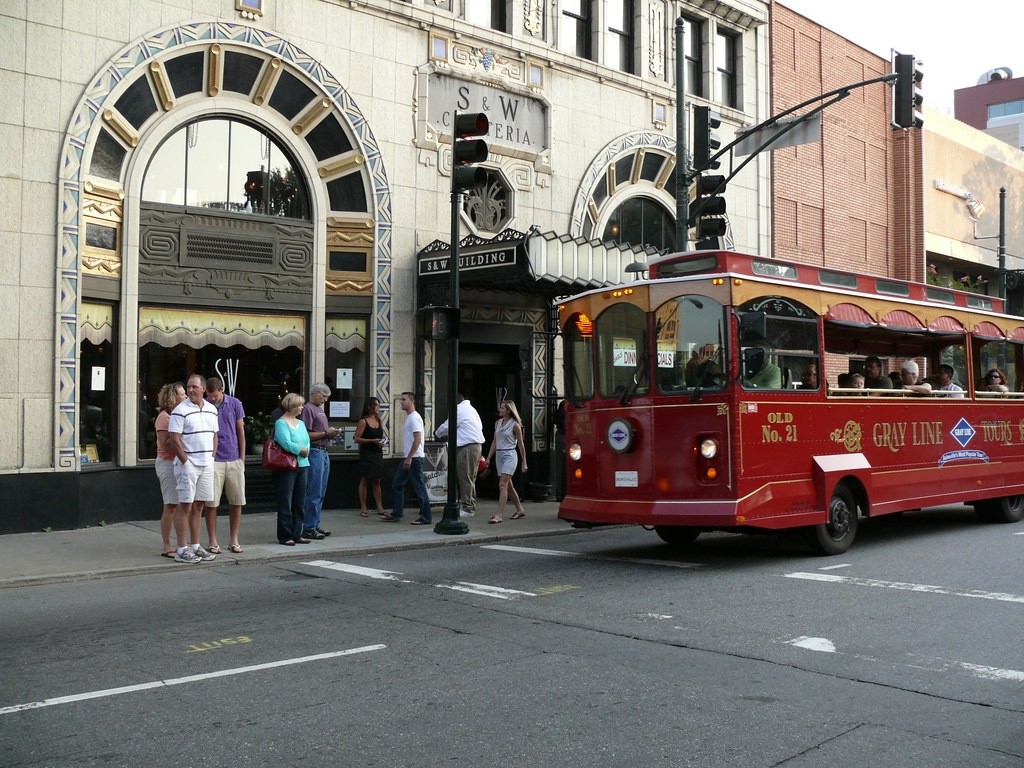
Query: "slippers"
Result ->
[[360, 510, 369, 517], [208, 543, 222, 553], [377, 510, 389, 516], [489, 517, 502, 523], [512, 511, 525, 519], [228, 544, 241, 552], [161, 549, 175, 558]]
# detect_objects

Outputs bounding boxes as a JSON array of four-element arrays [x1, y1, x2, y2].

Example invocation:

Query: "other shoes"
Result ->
[[294, 537, 310, 544], [380, 512, 400, 521], [280, 539, 294, 546], [411, 516, 429, 525], [458, 508, 473, 517]]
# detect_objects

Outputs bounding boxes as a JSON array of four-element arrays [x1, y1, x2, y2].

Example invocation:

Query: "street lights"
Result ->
[[965, 193, 1008, 368]]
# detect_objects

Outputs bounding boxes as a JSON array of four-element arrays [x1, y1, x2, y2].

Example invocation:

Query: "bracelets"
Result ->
[[486, 456, 491, 460], [752, 383, 758, 388], [325, 431, 328, 438]]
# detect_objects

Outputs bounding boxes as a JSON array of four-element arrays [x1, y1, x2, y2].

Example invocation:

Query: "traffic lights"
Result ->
[[449, 110, 488, 193], [891, 54, 925, 131], [693, 106, 722, 170], [696, 173, 727, 240]]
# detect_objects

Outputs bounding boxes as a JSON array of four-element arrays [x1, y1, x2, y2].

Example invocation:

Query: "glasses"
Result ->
[[989, 374, 1000, 379]]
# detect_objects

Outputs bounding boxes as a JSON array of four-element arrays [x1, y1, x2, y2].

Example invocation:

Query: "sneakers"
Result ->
[[318, 527, 331, 536], [302, 529, 325, 540], [175, 547, 202, 564], [191, 545, 216, 561]]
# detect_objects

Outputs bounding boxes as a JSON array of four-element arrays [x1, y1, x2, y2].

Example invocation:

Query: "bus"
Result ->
[[553, 249, 1024, 557]]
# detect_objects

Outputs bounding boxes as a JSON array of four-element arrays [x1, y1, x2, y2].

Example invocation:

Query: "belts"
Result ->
[[310, 446, 328, 451]]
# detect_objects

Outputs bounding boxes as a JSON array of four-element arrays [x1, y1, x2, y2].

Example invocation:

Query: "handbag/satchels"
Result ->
[[262, 421, 299, 473]]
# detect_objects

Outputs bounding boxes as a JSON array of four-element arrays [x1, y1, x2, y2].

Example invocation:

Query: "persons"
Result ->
[[931, 364, 964, 398], [378, 392, 432, 525], [433, 393, 486, 518], [833, 355, 937, 398], [154, 382, 188, 558], [274, 393, 312, 546], [554, 399, 567, 502], [168, 373, 219, 563], [485, 400, 528, 524], [299, 382, 340, 540], [977, 369, 1009, 399], [354, 397, 390, 517], [862, 355, 893, 397], [797, 363, 829, 396], [891, 361, 933, 397], [847, 373, 865, 396], [204, 378, 246, 554], [743, 339, 782, 389]]
